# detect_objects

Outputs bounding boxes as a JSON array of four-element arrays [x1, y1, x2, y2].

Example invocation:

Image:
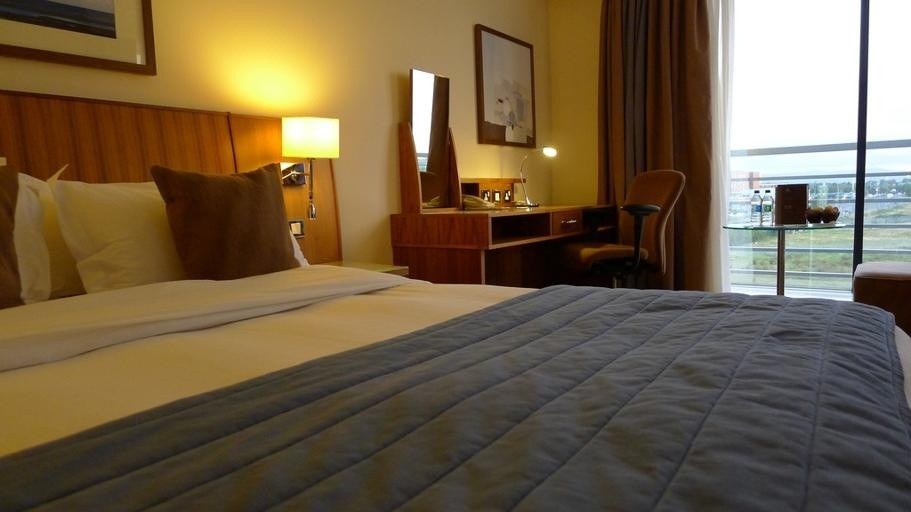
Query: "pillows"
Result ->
[[11, 160, 302, 305]]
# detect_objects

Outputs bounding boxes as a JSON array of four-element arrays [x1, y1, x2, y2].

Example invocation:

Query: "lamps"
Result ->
[[517, 144, 557, 207], [277, 114, 342, 220]]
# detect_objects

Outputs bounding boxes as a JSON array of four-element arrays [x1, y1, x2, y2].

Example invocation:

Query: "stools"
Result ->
[[851, 261, 911, 338]]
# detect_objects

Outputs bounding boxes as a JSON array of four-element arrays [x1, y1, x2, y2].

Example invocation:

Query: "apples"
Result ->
[[815, 207, 824, 213], [825, 205, 833, 215]]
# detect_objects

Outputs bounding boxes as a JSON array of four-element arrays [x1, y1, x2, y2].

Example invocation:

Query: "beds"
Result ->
[[0, 91, 908, 512]]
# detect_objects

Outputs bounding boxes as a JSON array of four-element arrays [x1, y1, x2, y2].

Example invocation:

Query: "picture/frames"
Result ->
[[473, 22, 537, 150], [0, 0, 158, 79]]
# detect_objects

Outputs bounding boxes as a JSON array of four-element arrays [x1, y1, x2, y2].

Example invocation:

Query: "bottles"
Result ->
[[762, 190, 773, 227], [750, 190, 763, 226]]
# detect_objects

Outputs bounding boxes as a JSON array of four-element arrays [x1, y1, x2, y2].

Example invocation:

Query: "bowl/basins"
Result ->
[[807, 213, 823, 223], [821, 212, 840, 223]]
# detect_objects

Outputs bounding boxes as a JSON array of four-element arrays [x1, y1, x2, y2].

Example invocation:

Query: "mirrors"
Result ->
[[409, 66, 453, 210]]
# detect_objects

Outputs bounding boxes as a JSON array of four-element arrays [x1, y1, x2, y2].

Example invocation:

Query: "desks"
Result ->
[[387, 205, 618, 286], [720, 221, 849, 295]]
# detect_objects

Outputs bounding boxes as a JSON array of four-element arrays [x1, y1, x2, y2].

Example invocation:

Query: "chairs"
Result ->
[[563, 167, 688, 289]]
